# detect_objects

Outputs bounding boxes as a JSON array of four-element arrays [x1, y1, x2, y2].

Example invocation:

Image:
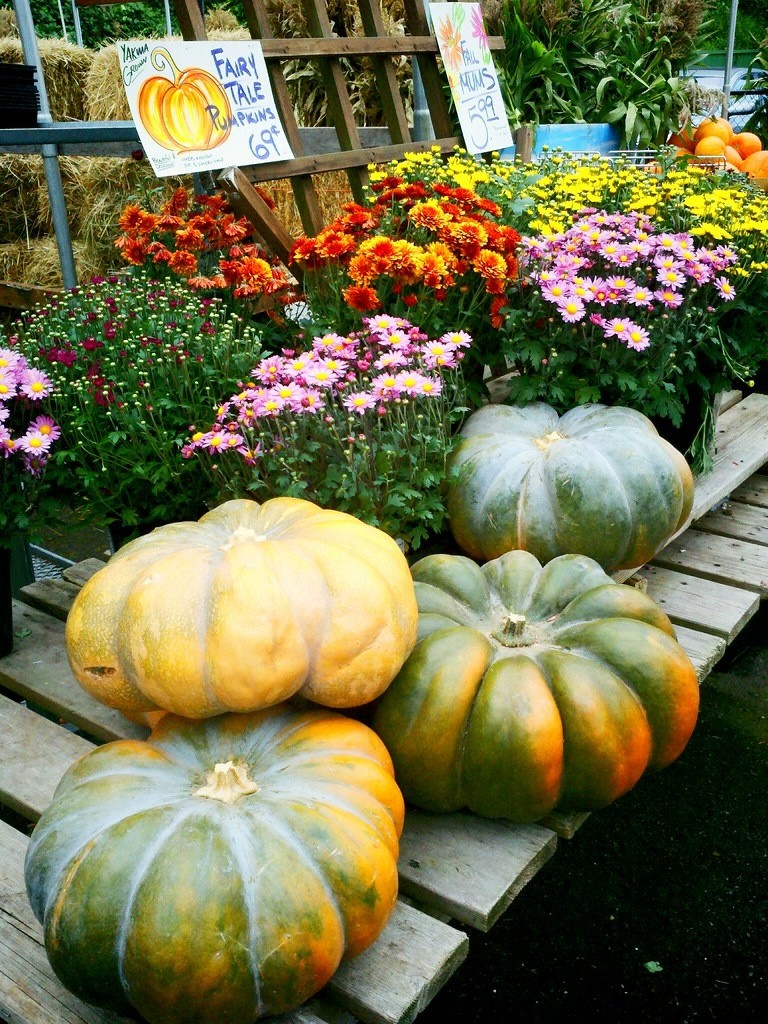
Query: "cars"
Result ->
[[672, 65, 768, 146]]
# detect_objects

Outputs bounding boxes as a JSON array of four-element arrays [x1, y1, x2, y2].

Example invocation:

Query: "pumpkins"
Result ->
[[352, 549, 701, 823], [446, 401, 694, 575], [22, 699, 404, 1024], [643, 114, 768, 193], [67, 494, 418, 731]]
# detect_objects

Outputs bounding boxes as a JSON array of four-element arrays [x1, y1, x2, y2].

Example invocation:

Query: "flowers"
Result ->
[[0, 137, 768, 558]]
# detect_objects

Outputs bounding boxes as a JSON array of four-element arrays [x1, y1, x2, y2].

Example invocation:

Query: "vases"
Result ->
[[406, 520, 462, 565], [67, 461, 212, 551], [0, 544, 13, 659], [731, 357, 765, 398], [649, 350, 714, 472]]
